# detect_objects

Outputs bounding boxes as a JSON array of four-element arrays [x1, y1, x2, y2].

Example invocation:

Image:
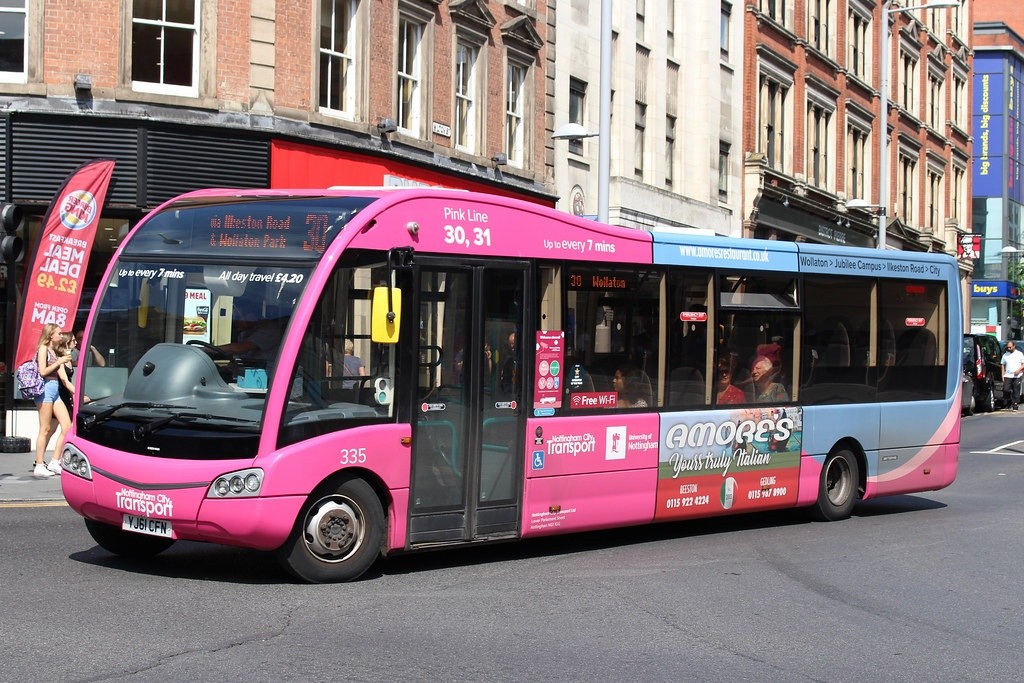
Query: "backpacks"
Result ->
[[16, 348, 45, 399]]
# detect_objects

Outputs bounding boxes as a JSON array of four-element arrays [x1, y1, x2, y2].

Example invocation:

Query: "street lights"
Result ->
[[845, 197, 886, 248], [879, 1, 961, 248], [551, 122, 611, 226]]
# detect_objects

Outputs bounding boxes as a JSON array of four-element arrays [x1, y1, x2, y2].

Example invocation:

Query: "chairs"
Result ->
[[485, 317, 942, 408]]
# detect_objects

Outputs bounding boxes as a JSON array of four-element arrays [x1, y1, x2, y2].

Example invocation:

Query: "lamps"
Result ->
[[869, 228, 878, 238], [832, 214, 842, 226], [379, 119, 399, 133], [75, 74, 91, 90], [491, 153, 508, 165], [778, 193, 790, 206], [839, 217, 851, 228]]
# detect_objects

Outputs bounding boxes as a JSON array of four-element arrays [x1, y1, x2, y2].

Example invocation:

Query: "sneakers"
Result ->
[[33, 464, 55, 476], [1013, 405, 1018, 411], [47, 458, 63, 475]]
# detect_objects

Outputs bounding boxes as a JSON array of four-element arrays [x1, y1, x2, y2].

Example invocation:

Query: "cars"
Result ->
[[962, 334, 1024, 412]]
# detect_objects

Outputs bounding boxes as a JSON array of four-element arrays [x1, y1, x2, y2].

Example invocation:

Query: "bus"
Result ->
[[61, 187, 962, 584]]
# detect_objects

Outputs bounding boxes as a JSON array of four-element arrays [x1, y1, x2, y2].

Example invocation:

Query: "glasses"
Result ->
[[719, 369, 730, 375], [71, 339, 77, 343]]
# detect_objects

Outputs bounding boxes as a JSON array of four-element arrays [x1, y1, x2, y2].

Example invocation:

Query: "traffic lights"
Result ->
[[0, 201, 26, 264]]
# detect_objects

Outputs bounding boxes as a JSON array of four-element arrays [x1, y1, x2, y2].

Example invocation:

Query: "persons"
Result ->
[[332, 339, 367, 389], [31, 324, 105, 477], [457, 316, 790, 407], [198, 301, 284, 380], [1000, 341, 1024, 410]]
